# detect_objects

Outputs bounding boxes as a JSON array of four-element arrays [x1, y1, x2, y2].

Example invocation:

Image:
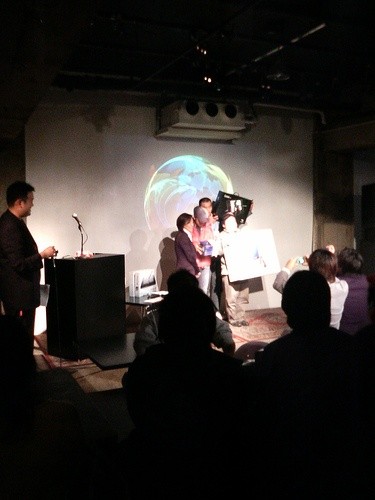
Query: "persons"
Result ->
[[126, 269, 375, 500], [0, 179, 56, 408], [175, 198, 250, 326], [273, 249, 349, 329], [326, 244, 375, 335]]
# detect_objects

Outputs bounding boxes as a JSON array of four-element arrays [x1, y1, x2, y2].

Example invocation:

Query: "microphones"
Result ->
[[72, 213, 82, 226]]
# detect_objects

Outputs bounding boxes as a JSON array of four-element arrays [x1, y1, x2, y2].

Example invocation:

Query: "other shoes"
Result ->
[[232, 320, 249, 327]]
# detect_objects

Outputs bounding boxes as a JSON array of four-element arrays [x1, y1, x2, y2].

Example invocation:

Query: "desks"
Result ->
[[125, 293, 164, 330]]
